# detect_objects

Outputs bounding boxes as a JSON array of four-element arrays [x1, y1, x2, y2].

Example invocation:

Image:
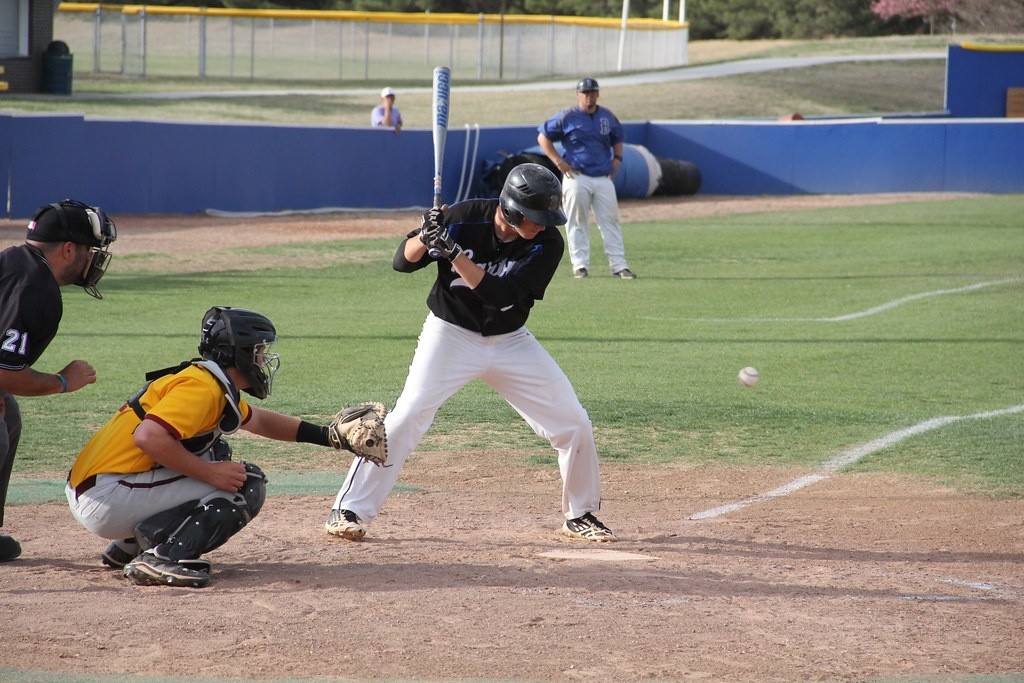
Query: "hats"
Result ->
[[381, 87, 395, 97], [27, 199, 117, 247]]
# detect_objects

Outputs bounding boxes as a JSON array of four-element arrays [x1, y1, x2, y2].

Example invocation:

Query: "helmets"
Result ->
[[197, 306, 281, 400], [498, 163, 568, 228], [577, 78, 600, 93]]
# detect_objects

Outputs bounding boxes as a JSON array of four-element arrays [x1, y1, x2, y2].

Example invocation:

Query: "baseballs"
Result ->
[[738, 366, 758, 388]]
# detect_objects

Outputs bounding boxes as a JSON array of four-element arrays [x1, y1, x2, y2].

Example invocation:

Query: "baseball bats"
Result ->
[[428, 65, 451, 259]]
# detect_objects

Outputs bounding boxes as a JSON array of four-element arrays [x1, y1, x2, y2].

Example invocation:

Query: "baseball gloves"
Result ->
[[327, 400, 390, 466]]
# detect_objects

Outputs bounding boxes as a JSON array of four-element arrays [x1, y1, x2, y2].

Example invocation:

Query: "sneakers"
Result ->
[[612, 268, 637, 279], [562, 513, 617, 541], [100, 540, 144, 570], [574, 269, 588, 278], [325, 508, 366, 540], [124, 551, 212, 585]]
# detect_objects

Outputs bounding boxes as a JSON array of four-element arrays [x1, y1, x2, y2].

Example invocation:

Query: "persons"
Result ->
[[64, 307, 389, 588], [538, 79, 636, 278], [325, 163, 616, 541], [0, 201, 118, 563], [372, 88, 402, 133]]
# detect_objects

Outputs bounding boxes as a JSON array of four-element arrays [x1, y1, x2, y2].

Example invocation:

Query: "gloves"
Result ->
[[419, 222, 461, 262], [420, 204, 449, 230]]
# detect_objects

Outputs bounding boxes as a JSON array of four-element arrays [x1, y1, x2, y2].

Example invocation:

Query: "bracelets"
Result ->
[[57, 374, 67, 393], [614, 155, 623, 161]]
